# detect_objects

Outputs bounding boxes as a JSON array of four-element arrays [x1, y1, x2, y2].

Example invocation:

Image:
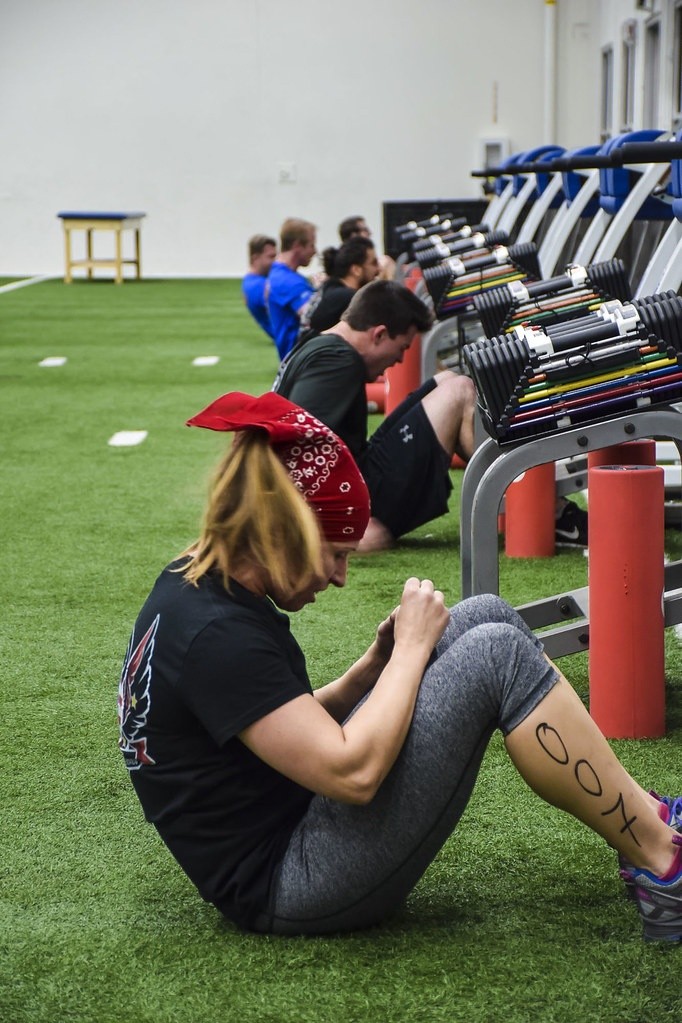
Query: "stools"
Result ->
[[57, 209, 146, 285]]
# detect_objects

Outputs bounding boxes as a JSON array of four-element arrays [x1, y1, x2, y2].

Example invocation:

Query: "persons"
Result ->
[[118, 393, 682, 936], [245, 212, 383, 353], [275, 281, 588, 556]]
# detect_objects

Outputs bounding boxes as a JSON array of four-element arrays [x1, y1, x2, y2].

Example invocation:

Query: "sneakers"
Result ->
[[618, 791, 682, 946], [553, 496, 589, 548]]
